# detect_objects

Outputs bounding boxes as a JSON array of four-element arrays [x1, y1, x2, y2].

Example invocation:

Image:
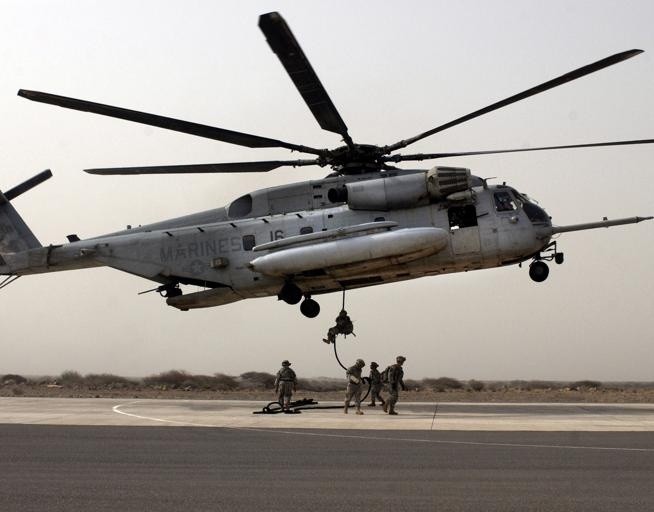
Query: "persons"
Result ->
[[367, 361, 385, 406], [273, 359, 297, 411], [344, 359, 365, 415], [323, 311, 353, 344], [494, 192, 514, 211], [382, 356, 408, 415]]
